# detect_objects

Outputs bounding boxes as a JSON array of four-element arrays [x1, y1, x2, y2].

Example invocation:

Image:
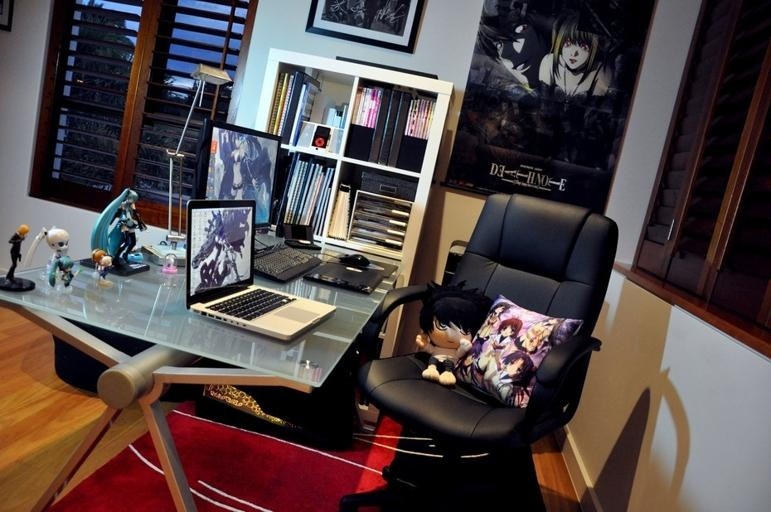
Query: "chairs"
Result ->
[[338, 193, 618, 512]]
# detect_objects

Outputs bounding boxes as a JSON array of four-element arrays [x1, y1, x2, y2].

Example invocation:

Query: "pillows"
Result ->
[[456, 296, 584, 409]]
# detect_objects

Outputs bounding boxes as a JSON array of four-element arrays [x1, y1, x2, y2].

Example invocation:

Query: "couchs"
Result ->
[[51, 317, 201, 403]]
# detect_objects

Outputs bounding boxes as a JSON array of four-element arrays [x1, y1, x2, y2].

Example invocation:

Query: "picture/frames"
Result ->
[[305, 0, 428, 55], [0, 0, 14, 33]]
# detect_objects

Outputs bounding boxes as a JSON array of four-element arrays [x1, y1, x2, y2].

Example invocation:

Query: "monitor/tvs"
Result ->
[[193, 117, 283, 230]]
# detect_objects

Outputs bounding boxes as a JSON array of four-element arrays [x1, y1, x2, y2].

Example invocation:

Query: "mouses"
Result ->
[[338, 254, 370, 267]]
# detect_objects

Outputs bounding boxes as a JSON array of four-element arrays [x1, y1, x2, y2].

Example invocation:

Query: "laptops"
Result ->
[[303, 263, 384, 294], [186, 199, 337, 342]]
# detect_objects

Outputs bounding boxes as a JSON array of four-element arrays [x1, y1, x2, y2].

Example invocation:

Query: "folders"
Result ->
[[368, 89, 393, 164], [351, 227, 404, 251], [354, 200, 410, 223], [351, 220, 406, 238], [352, 211, 407, 231], [377, 88, 403, 167], [387, 91, 413, 169], [327, 183, 351, 241], [355, 194, 412, 209]]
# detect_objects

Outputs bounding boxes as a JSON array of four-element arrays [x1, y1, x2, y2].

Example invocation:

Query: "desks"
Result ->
[[0, 247, 400, 511]]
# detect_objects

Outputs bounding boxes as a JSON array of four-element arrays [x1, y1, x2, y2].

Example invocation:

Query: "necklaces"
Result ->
[[564, 64, 587, 113]]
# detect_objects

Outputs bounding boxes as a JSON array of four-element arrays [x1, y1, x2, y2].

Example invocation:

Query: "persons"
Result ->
[[24, 226, 75, 288], [5, 225, 30, 283], [416, 281, 487, 386], [477, 0, 546, 92], [451, 302, 566, 406], [89, 188, 146, 268], [49, 253, 75, 288], [534, 11, 613, 134], [218, 130, 271, 218]]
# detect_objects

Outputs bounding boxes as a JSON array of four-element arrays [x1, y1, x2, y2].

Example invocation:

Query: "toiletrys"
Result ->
[[340, 103, 348, 129], [333, 110, 343, 127], [326, 105, 337, 126]]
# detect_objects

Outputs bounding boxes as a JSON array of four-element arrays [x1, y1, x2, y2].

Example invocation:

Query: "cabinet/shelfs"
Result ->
[[253, 45, 454, 424]]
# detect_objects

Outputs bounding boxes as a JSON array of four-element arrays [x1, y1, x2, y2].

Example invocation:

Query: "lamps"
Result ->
[[141, 63, 232, 266]]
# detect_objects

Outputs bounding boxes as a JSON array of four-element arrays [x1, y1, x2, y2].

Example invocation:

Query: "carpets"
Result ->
[[46, 400, 403, 512]]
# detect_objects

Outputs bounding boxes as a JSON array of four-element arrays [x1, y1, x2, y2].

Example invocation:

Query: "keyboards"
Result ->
[[253, 246, 323, 284]]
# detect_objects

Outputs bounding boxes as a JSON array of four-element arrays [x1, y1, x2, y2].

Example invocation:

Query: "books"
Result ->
[[278, 157, 337, 241], [80, 258, 149, 276], [344, 80, 437, 173], [268, 63, 320, 146], [329, 166, 415, 256]]
